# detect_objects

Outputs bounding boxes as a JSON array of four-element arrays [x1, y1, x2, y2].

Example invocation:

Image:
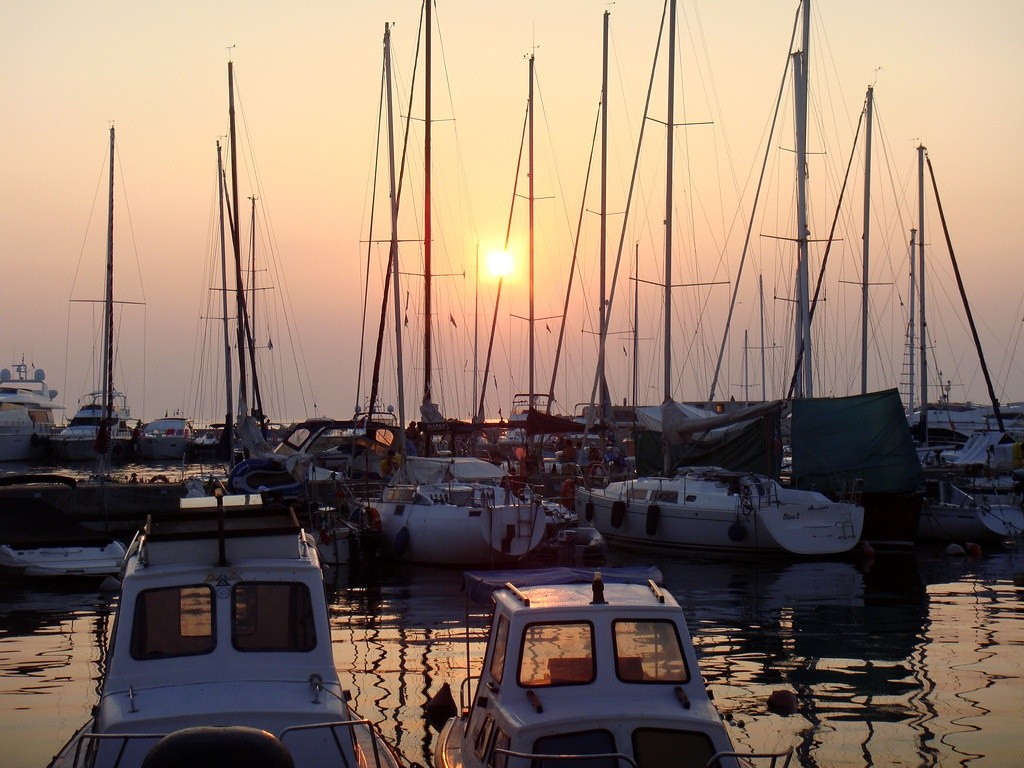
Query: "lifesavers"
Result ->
[[370, 509, 383, 537], [560, 479, 576, 507]]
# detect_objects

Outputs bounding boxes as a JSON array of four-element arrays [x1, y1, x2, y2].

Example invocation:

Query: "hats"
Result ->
[[590, 442, 597, 446]]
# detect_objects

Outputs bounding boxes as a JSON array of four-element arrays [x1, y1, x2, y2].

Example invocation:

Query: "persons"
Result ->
[[399, 432, 417, 457], [562, 439, 588, 465], [933, 449, 945, 463], [378, 446, 402, 483], [587, 441, 602, 462]]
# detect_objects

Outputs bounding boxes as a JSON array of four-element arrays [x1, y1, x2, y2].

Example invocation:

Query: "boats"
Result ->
[[45, 489, 407, 768], [433, 571, 794, 768], [0, 367, 58, 458]]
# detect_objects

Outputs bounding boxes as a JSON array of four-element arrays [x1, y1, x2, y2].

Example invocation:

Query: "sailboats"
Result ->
[[54, 0, 1023, 563]]
